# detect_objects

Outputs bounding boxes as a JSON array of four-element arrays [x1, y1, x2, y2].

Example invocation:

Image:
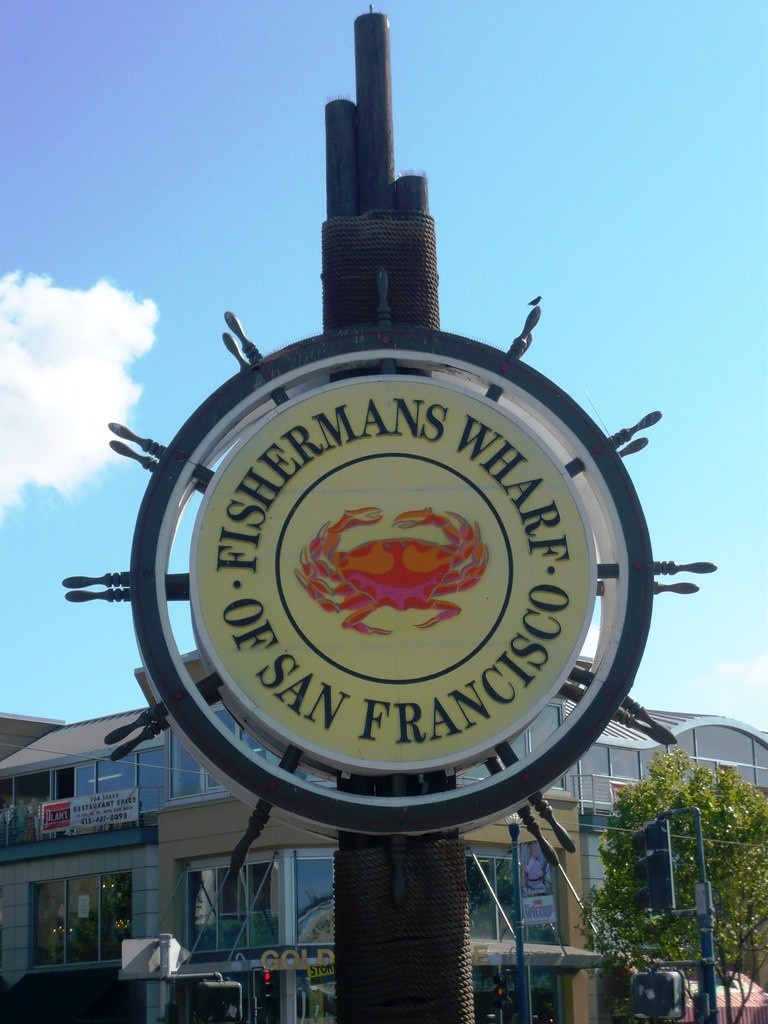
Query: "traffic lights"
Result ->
[[260, 969, 272, 1003], [493, 973, 508, 1010], [631, 821, 676, 910], [195, 981, 242, 1022]]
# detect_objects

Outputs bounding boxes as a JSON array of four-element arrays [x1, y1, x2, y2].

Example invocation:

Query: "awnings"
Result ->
[[615, 953, 768, 1024], [0, 967, 121, 1024]]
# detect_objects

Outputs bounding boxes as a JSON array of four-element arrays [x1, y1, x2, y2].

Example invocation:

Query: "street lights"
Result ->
[[506, 810, 535, 1024]]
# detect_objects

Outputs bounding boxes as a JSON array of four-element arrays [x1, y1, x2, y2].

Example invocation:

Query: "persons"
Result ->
[[526, 843, 550, 889]]
